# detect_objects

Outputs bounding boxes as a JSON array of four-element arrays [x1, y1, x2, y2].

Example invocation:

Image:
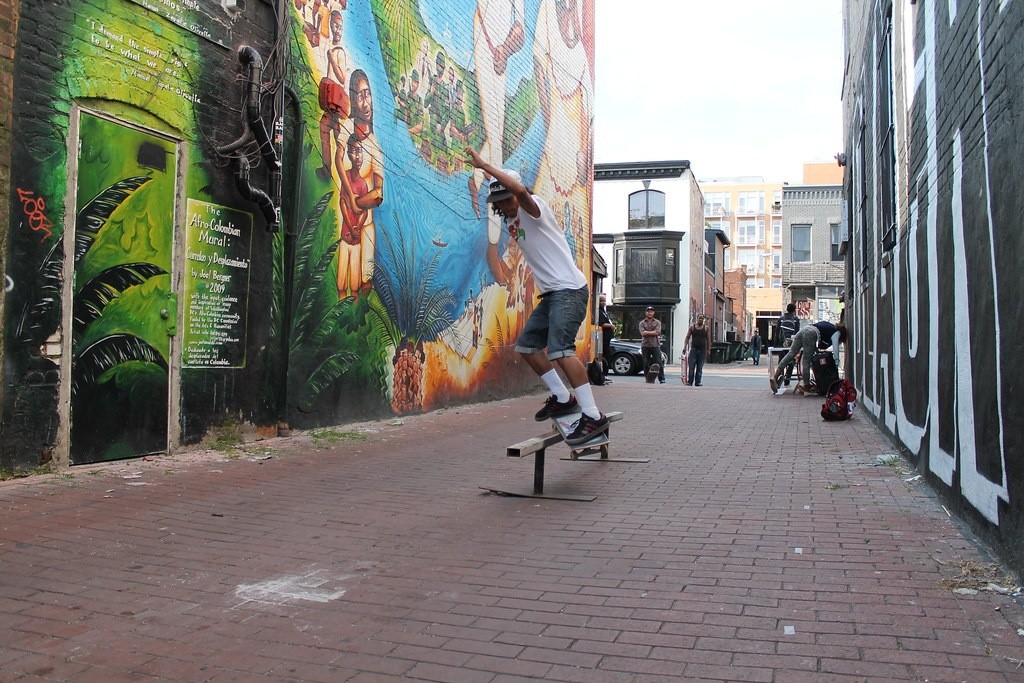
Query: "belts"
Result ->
[[537, 291, 553, 299]]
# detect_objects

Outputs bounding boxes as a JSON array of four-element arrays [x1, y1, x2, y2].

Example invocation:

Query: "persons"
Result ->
[[638, 306, 666, 384], [769, 319, 849, 393], [683, 314, 711, 386], [599, 295, 616, 385], [774, 302, 800, 388], [751, 329, 762, 365], [462, 146, 610, 444]]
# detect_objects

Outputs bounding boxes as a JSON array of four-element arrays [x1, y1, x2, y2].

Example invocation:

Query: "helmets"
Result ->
[[490, 169, 521, 194]]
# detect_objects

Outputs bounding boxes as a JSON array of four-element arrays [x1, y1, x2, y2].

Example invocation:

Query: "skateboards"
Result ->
[[646, 363, 660, 383], [552, 412, 611, 461], [678, 351, 688, 385]]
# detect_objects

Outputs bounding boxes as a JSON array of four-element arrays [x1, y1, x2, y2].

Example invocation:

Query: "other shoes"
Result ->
[[784, 384, 790, 387], [777, 375, 784, 389]]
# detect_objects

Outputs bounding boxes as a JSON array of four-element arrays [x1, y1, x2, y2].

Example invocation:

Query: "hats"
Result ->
[[486, 192, 513, 203], [646, 306, 655, 311]]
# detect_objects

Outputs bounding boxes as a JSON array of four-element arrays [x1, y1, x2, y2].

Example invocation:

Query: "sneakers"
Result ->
[[809, 387, 815, 391], [604, 376, 612, 383], [695, 382, 703, 386], [535, 394, 579, 422], [689, 381, 692, 386], [565, 411, 610, 445], [770, 376, 777, 393]]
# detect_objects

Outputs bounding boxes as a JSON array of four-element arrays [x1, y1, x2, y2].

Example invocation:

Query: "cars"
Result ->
[[608, 338, 668, 376]]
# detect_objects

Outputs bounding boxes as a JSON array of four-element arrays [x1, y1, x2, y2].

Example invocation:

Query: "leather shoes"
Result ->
[[660, 380, 666, 384]]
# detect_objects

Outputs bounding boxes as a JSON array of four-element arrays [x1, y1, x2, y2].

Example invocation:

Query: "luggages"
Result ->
[[812, 351, 839, 395]]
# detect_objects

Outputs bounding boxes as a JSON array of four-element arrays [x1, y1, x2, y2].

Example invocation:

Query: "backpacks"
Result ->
[[779, 319, 796, 343], [821, 379, 857, 421]]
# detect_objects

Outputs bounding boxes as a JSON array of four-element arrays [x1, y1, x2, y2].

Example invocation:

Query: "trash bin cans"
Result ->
[[711, 342, 732, 363], [730, 340, 744, 361]]
